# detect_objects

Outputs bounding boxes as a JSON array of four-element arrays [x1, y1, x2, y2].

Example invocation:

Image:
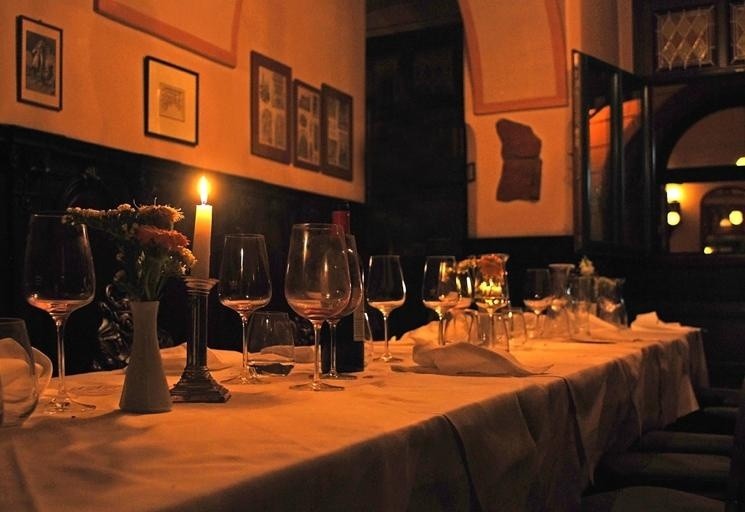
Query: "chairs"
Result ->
[[580, 319, 743, 511]]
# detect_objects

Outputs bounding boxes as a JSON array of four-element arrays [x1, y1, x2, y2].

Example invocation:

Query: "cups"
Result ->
[[0, 318, 38, 431], [481, 309, 569, 348], [247, 312, 295, 379]]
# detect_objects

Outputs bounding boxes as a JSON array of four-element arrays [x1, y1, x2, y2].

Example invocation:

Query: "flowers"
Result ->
[[66, 191, 197, 301]]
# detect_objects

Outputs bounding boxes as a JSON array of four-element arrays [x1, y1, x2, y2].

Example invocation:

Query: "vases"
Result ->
[[118, 300, 175, 412]]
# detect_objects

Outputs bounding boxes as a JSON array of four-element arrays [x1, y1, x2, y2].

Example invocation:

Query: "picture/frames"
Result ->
[[247, 48, 356, 184], [16, 15, 64, 111], [142, 55, 201, 148]]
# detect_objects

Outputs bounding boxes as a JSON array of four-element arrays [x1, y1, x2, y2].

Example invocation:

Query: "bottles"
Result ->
[[320, 201, 365, 376]]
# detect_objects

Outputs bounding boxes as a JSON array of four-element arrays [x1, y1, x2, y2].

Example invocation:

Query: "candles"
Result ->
[[189, 174, 215, 278]]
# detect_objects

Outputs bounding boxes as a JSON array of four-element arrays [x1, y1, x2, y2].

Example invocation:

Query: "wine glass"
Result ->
[[362, 255, 408, 366], [284, 225, 353, 393], [421, 255, 512, 349], [316, 248, 367, 379], [519, 265, 631, 343], [218, 231, 271, 386], [25, 214, 96, 416]]
